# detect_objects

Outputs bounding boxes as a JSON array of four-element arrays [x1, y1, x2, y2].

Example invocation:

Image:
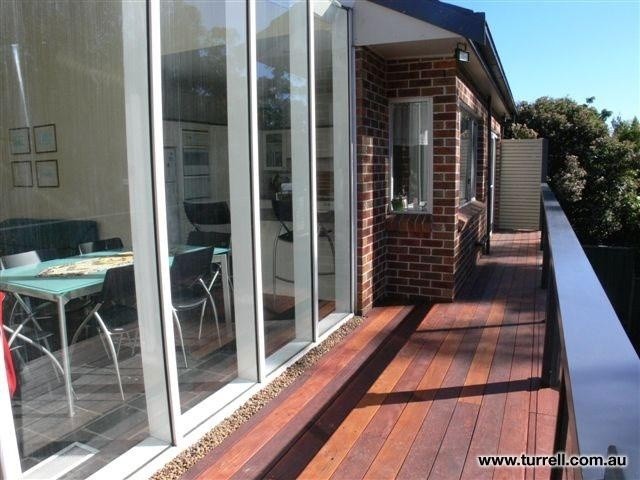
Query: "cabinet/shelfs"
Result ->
[[263, 127, 333, 171]]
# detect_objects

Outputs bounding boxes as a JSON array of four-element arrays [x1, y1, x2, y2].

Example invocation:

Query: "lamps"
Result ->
[[454, 42, 470, 62]]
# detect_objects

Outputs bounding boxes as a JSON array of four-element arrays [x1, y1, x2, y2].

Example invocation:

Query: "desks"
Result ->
[[0, 243, 233, 419]]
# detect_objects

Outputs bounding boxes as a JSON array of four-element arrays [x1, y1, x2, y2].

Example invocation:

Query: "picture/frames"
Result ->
[[8, 127, 31, 155], [35, 159, 59, 188], [10, 161, 33, 188], [33, 124, 57, 153]]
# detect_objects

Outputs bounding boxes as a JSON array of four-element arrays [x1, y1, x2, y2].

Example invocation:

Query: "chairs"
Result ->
[[78, 236, 137, 360], [3, 289, 79, 401], [0, 249, 91, 384], [269, 197, 335, 314], [140, 244, 221, 367], [185, 230, 230, 340], [68, 264, 138, 401], [182, 201, 231, 231]]
